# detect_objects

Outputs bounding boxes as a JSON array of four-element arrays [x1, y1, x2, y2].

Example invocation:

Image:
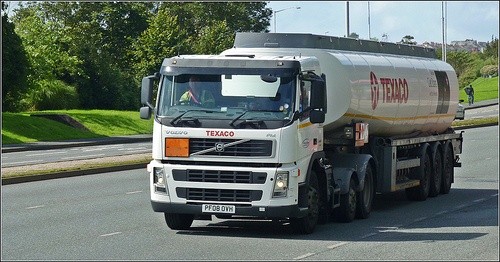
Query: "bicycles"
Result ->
[[466, 93, 476, 106]]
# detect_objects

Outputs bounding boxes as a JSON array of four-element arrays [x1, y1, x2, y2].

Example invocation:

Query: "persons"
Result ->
[[179, 75, 215, 108], [467, 85, 474, 106]]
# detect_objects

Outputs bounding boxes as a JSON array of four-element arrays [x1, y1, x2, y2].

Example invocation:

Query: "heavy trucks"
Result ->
[[140, 33, 463, 234]]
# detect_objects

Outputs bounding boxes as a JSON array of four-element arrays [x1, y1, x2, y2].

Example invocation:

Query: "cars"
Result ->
[[454, 98, 465, 120]]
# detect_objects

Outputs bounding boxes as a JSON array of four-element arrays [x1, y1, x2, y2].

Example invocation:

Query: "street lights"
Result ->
[[274, 5, 301, 32]]
